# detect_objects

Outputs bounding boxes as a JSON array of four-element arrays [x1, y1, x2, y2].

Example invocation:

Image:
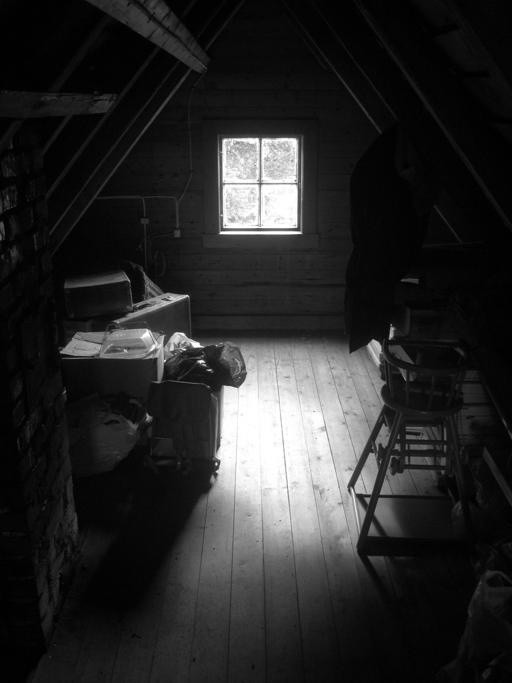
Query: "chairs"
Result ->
[[349, 336, 482, 561]]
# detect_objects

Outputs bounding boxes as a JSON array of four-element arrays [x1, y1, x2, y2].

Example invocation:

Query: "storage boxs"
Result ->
[[58, 327, 167, 405], [62, 263, 134, 321], [149, 378, 220, 459], [389, 280, 474, 340]]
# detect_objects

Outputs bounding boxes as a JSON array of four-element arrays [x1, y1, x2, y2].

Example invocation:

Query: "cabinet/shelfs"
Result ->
[[452, 348, 511, 600]]
